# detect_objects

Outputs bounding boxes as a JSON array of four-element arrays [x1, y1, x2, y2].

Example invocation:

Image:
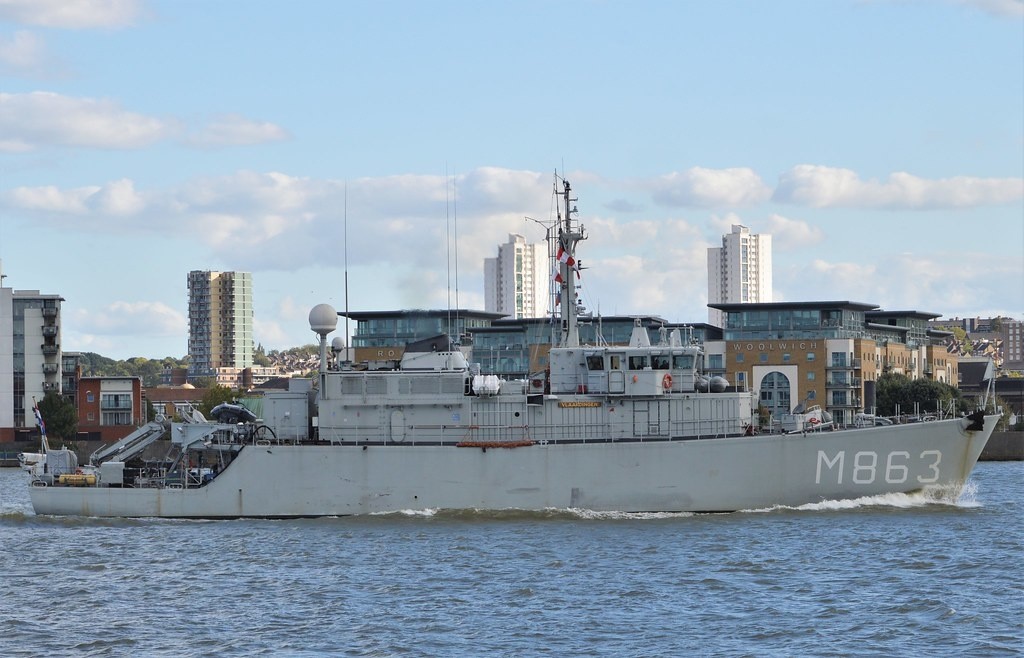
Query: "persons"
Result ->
[[660, 360, 669, 369]]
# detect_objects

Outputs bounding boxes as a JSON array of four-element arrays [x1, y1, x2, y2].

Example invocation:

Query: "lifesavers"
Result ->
[[664, 373, 673, 388], [181, 459, 193, 471]]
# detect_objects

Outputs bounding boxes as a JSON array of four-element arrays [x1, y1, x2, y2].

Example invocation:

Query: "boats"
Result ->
[[18, 165, 1004, 519]]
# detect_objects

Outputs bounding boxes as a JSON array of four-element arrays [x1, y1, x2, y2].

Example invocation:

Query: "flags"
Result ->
[[553, 268, 562, 282], [556, 248, 580, 279], [556, 293, 561, 306]]
